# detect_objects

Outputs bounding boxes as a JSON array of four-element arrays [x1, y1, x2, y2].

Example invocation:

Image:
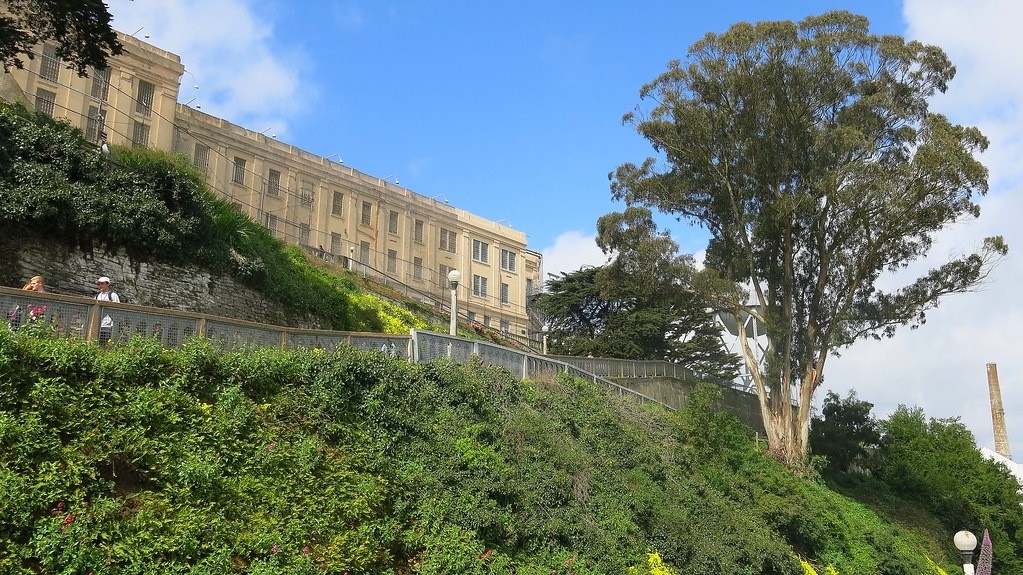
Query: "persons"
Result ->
[[92, 277, 121, 345], [18, 276, 50, 318]]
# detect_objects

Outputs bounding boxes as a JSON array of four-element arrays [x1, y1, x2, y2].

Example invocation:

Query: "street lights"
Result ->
[[541, 326, 549, 355], [953, 530, 978, 575], [446, 269, 462, 336]]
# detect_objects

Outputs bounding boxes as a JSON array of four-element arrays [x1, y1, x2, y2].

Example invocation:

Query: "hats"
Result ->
[[96, 276, 110, 285]]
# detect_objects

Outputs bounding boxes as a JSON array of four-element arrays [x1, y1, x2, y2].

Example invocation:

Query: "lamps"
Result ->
[[130, 27, 149, 38], [185, 97, 202, 108], [382, 174, 399, 184], [498, 218, 513, 228], [259, 127, 277, 138], [184, 69, 199, 88], [432, 193, 449, 203], [326, 152, 343, 164]]
